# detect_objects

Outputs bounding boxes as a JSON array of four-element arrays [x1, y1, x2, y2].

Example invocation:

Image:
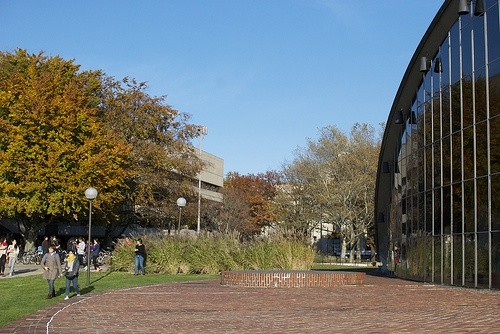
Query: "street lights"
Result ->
[[177, 196, 186, 235], [84, 186, 98, 286]]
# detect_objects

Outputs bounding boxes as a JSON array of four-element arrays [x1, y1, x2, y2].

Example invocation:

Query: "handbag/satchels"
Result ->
[[65, 272, 75, 280]]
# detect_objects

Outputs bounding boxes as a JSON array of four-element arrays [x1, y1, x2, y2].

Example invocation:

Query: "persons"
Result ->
[[134, 239, 147, 275], [64, 250, 80, 300], [0, 235, 60, 276], [41, 244, 63, 298], [75, 237, 100, 271]]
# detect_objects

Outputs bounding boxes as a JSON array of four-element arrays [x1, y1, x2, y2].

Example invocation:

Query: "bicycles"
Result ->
[[15, 251, 44, 265]]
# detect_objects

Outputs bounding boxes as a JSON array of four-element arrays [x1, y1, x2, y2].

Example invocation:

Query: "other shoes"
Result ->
[[134, 273, 138, 275], [10, 273, 12, 276], [48, 294, 55, 298], [64, 295, 69, 300], [141, 273, 145, 275], [0, 273, 3, 275], [77, 294, 80, 296], [2, 272, 5, 277]]
